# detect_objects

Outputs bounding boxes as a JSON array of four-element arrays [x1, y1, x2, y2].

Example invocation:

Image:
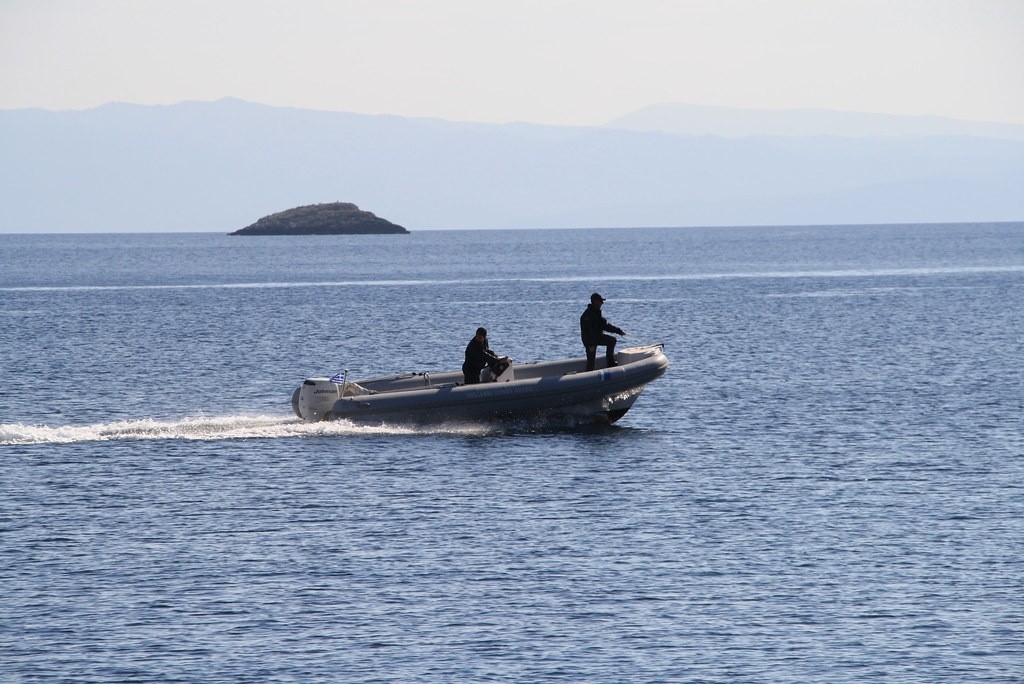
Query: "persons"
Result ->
[[462, 327, 509, 385], [580, 293, 626, 372]]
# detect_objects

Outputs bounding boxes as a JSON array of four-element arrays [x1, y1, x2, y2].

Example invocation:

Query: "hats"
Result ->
[[476, 327, 487, 337], [591, 293, 606, 300]]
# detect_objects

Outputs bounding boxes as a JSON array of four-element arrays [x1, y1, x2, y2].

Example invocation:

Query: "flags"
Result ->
[[329, 373, 345, 385]]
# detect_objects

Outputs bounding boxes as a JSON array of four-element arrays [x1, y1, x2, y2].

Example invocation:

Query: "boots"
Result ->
[[605, 352, 617, 366]]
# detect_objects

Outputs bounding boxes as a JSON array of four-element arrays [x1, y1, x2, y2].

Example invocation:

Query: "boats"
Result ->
[[293, 343, 671, 429]]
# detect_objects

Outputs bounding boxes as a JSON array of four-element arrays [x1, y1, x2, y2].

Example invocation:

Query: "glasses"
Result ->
[[597, 301, 604, 303]]
